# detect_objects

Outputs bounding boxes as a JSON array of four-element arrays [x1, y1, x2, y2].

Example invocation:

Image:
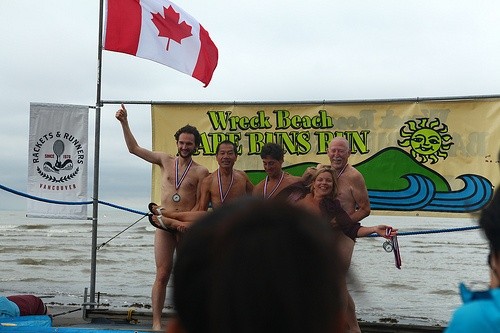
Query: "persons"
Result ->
[[148, 169, 398, 239], [445, 182, 500, 333], [177, 140, 252, 232], [167, 199, 362, 333], [253, 142, 300, 200], [318, 137, 370, 222], [117, 104, 209, 331]]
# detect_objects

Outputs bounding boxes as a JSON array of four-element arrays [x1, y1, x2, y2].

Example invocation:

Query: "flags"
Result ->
[[102, 0, 219, 88]]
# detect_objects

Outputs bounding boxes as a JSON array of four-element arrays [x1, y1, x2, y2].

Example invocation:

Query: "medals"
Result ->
[[384, 241, 393, 252], [173, 193, 181, 202]]
